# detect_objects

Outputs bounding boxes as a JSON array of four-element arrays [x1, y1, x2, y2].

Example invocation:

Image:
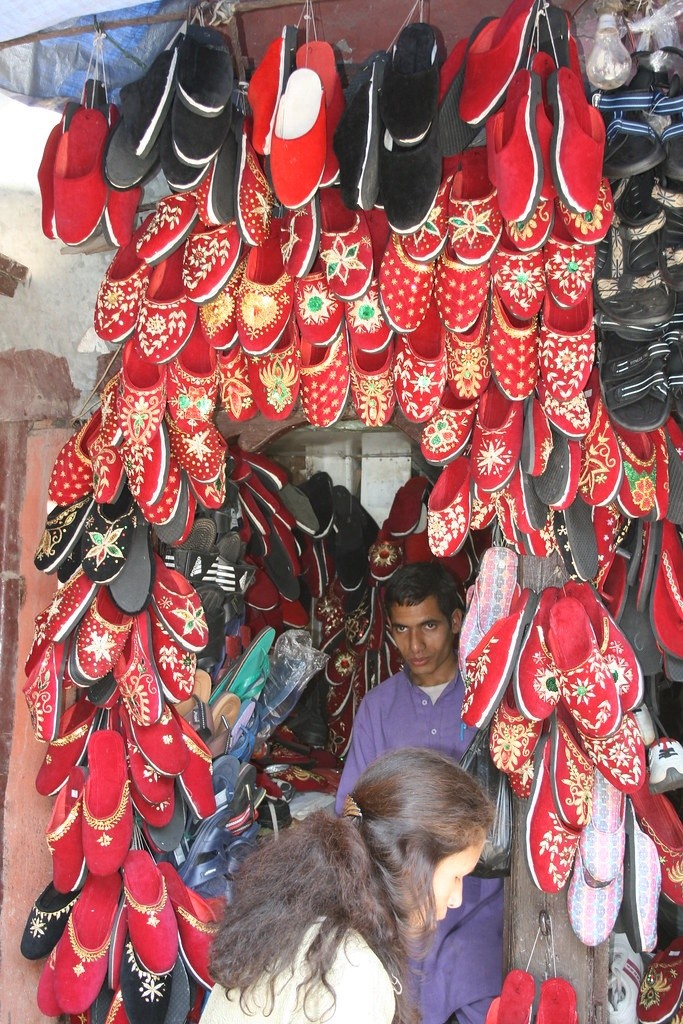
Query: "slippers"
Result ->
[[20, 0, 683, 1024]]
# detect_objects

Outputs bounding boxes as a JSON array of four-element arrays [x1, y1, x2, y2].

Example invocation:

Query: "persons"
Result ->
[[201, 746, 495, 1024], [334, 563, 503, 1024]]
